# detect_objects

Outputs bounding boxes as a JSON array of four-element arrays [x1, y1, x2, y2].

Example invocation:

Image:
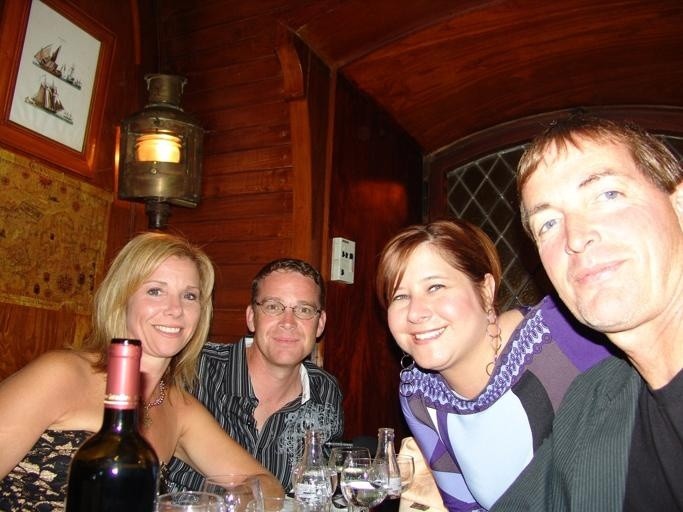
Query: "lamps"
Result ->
[[117, 59, 205, 232]]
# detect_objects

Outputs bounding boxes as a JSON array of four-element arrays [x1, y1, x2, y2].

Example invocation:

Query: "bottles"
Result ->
[[290, 425, 414, 511], [65, 338, 160, 511]]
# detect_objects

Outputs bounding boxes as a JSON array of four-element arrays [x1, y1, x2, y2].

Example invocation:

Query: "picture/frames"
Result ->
[[0, 0, 119, 181]]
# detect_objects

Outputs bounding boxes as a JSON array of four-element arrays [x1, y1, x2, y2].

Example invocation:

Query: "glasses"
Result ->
[[253, 299, 322, 320]]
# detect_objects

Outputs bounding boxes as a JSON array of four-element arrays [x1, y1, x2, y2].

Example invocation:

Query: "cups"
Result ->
[[159, 473, 304, 510]]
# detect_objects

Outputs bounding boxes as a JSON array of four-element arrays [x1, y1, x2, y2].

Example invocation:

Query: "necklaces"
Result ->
[[139, 382, 165, 433]]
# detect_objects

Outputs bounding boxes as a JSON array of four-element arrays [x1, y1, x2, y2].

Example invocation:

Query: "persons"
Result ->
[[376, 218, 612, 512], [1, 230, 286, 511], [484, 111, 683, 512], [159, 258, 345, 504]]
